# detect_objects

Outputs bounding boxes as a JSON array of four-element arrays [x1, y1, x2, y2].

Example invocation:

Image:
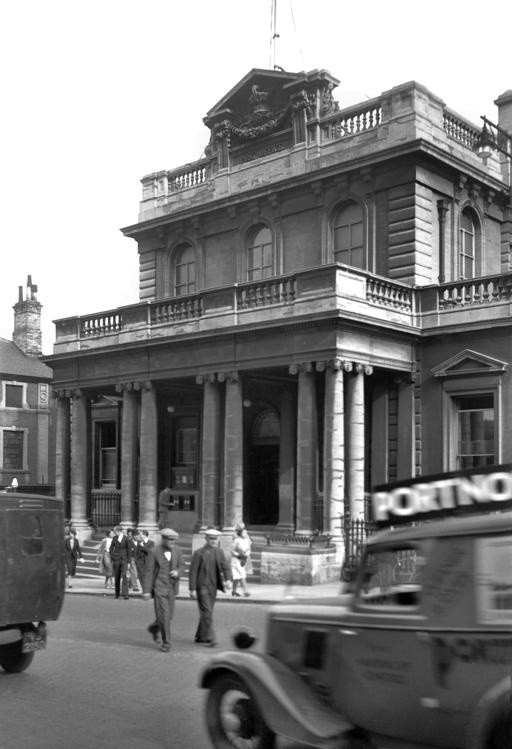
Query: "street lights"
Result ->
[[471, 116, 512, 166]]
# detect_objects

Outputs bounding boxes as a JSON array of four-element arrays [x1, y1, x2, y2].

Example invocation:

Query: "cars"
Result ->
[[0, 493, 65, 672], [196, 511, 512, 749]]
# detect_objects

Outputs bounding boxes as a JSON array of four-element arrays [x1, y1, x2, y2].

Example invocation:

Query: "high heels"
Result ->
[[232, 591, 251, 597]]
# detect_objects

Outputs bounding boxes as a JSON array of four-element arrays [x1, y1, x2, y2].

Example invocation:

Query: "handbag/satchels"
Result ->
[[238, 555, 247, 567]]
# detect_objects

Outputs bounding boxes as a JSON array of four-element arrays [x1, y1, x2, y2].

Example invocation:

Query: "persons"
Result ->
[[229, 528, 252, 598], [231, 522, 254, 588], [142, 527, 185, 653], [189, 528, 234, 647], [64, 528, 86, 589], [96, 525, 156, 600]]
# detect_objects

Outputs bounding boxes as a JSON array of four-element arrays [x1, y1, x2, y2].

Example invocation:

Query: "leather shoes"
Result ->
[[148, 625, 170, 652], [105, 583, 140, 600], [194, 634, 215, 643]]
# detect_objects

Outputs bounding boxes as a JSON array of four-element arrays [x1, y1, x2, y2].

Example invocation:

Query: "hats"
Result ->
[[235, 632, 255, 648], [159, 528, 179, 539], [205, 529, 221, 539]]
